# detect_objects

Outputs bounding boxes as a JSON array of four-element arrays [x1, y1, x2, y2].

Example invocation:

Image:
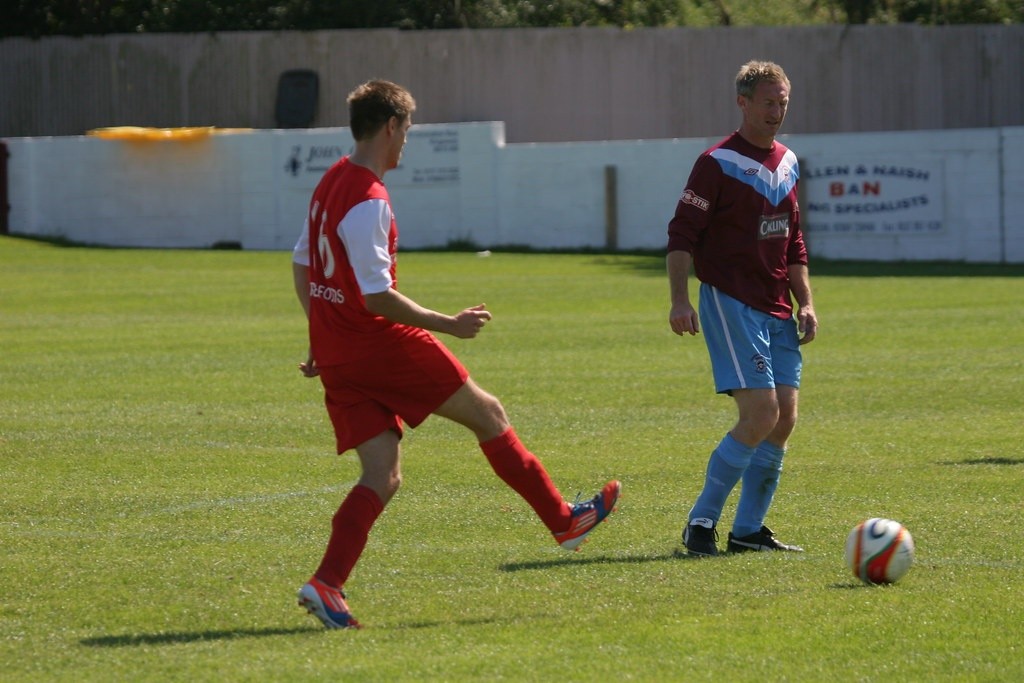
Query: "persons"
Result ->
[[665, 59, 817, 556], [294, 77, 623, 630]]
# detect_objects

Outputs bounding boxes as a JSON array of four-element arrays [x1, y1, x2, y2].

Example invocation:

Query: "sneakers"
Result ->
[[680, 521, 720, 559], [727, 525, 804, 558], [298, 574, 367, 632], [550, 480, 622, 550]]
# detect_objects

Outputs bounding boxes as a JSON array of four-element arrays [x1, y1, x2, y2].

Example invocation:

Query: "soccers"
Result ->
[[845, 517, 915, 585]]
